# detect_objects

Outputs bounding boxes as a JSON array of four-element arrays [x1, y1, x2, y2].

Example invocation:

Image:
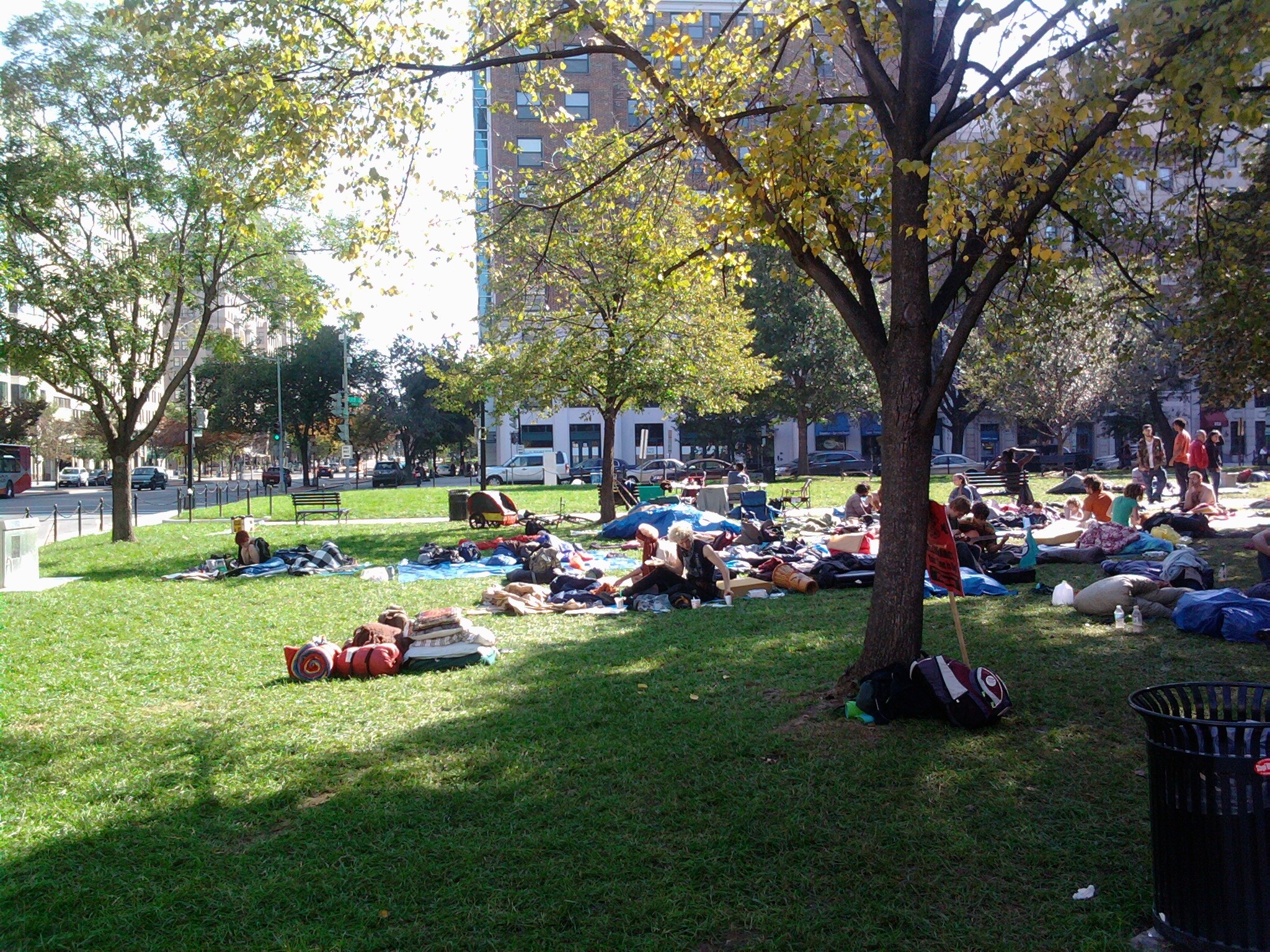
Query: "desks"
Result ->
[[680, 485, 730, 509]]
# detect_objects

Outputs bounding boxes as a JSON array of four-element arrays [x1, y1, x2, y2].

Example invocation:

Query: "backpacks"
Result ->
[[459, 541, 481, 562], [910, 650, 1011, 730], [856, 660, 910, 725]]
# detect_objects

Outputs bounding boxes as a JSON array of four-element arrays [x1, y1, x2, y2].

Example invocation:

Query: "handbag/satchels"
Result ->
[[1016, 481, 1035, 508]]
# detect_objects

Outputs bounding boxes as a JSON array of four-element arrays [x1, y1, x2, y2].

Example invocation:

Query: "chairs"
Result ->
[[725, 483, 750, 507], [391, 464, 396, 469], [739, 489, 782, 525], [780, 479, 814, 510], [697, 486, 730, 518]]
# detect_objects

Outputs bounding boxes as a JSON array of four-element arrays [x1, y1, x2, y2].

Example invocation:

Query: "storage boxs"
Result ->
[[229, 516, 253, 534]]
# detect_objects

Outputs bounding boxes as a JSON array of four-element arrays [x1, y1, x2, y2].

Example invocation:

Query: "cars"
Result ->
[[872, 461, 882, 476], [570, 458, 638, 484], [1094, 445, 1138, 471], [328, 463, 340, 473], [316, 465, 334, 478], [931, 448, 947, 460], [650, 458, 737, 483], [984, 444, 1070, 476], [436, 464, 451, 476], [89, 469, 107, 486], [930, 453, 985, 475], [775, 449, 868, 477], [106, 471, 113, 486], [626, 458, 696, 484], [131, 466, 168, 491]]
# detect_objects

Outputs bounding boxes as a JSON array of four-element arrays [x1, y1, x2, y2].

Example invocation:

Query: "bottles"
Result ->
[[1131, 605, 1143, 633], [1113, 605, 1125, 628], [1052, 580, 1074, 606]]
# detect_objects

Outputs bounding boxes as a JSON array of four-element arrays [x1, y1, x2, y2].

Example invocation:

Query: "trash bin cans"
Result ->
[[763, 464, 775, 482], [614, 466, 623, 484], [447, 489, 470, 521], [1129, 682, 1270, 952], [590, 473, 602, 485]]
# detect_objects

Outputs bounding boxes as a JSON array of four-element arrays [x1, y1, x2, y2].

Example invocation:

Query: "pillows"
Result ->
[[826, 532, 871, 555]]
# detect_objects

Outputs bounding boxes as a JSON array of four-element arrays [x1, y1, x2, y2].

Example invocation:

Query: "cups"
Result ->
[[724, 595, 732, 605], [614, 597, 623, 608], [691, 599, 701, 609]]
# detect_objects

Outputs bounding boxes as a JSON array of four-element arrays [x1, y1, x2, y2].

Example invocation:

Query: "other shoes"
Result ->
[[1148, 498, 1164, 505], [600, 592, 621, 604]]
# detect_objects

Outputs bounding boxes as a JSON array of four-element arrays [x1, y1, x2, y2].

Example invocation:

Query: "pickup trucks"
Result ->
[[262, 467, 292, 487]]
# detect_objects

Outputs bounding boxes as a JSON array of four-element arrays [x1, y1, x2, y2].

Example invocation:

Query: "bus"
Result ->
[[0, 443, 32, 499]]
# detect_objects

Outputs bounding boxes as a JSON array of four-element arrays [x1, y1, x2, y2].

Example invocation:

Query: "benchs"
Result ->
[[961, 469, 1031, 505], [596, 480, 660, 512], [291, 491, 353, 525]]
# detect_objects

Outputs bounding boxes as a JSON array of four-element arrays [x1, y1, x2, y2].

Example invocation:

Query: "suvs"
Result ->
[[366, 460, 404, 487], [476, 452, 571, 486], [58, 466, 90, 487]]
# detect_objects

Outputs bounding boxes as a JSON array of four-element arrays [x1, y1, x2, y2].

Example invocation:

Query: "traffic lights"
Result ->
[[337, 424, 346, 440], [273, 421, 280, 441], [330, 392, 342, 417], [509, 416, 518, 428]]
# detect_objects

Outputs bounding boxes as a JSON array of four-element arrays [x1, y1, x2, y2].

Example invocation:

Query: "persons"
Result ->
[[1168, 418, 1224, 505], [942, 473, 1015, 570], [728, 462, 751, 511], [612, 521, 733, 605], [1259, 446, 1267, 465], [1132, 424, 1167, 505], [1252, 526, 1270, 580], [984, 447, 1036, 503], [1183, 471, 1216, 510], [612, 523, 684, 589], [1067, 474, 1112, 522], [845, 480, 874, 518], [1027, 500, 1047, 533], [864, 486, 882, 514], [1106, 483, 1144, 530], [235, 531, 271, 566], [1119, 441, 1131, 470], [450, 459, 479, 476], [411, 462, 422, 487]]
